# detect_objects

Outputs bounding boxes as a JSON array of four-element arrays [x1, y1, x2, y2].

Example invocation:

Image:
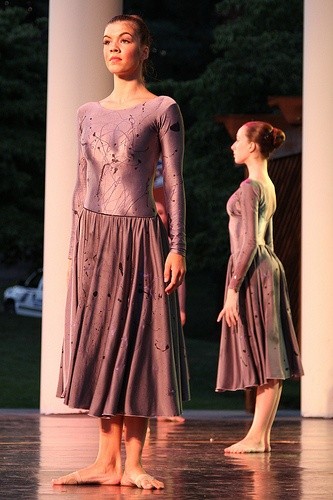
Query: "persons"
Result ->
[[215, 122, 304, 453], [52, 15, 190, 489]]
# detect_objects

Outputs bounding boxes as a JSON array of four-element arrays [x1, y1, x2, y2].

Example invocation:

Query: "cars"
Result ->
[[3, 270, 43, 318]]
